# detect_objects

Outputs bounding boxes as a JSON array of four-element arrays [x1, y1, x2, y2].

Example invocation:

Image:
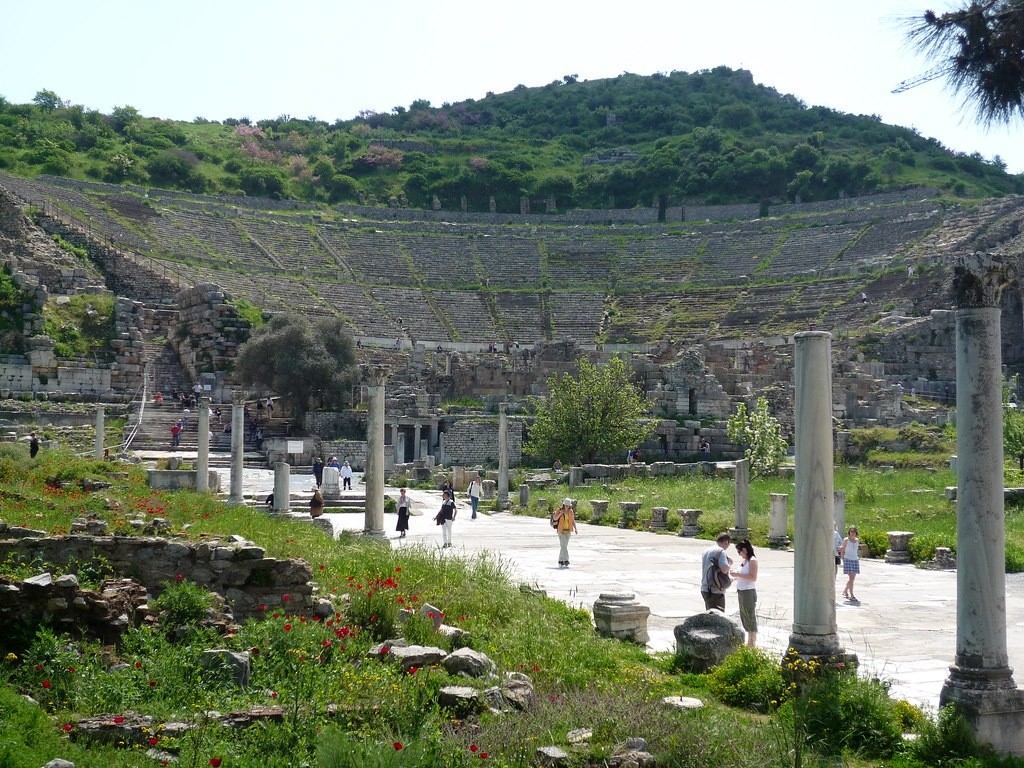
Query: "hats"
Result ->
[[311, 484, 319, 491], [332, 457, 337, 460], [563, 497, 573, 507]]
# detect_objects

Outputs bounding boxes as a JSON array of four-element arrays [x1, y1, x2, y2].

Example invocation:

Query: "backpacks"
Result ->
[[550, 507, 565, 529], [468, 480, 475, 493], [705, 548, 732, 592]]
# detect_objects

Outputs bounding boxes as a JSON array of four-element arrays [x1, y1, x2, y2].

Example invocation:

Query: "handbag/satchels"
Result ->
[[437, 509, 445, 525], [310, 495, 320, 507]]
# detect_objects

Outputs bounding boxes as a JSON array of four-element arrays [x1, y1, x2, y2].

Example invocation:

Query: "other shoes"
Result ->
[[558, 560, 564, 565], [349, 487, 352, 490], [443, 543, 447, 547], [344, 486, 347, 490], [448, 543, 452, 547], [565, 560, 570, 566], [400, 534, 405, 537]]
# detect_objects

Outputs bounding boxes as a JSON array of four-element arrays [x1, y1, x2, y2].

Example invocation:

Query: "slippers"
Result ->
[[842, 593, 849, 598], [848, 597, 858, 601]]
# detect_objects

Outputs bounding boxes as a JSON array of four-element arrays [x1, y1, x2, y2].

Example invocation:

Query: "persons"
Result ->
[[434, 476, 483, 548], [29, 433, 39, 458], [701, 532, 758, 647], [899, 382, 916, 397], [110, 234, 116, 246], [156, 383, 275, 448], [832, 521, 860, 601], [396, 488, 411, 538], [266, 456, 354, 520], [860, 292, 867, 304], [552, 439, 710, 471], [553, 498, 578, 566], [908, 265, 917, 276], [357, 317, 521, 353]]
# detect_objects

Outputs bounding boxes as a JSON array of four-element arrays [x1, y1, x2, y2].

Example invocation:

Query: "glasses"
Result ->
[[738, 550, 741, 554]]
